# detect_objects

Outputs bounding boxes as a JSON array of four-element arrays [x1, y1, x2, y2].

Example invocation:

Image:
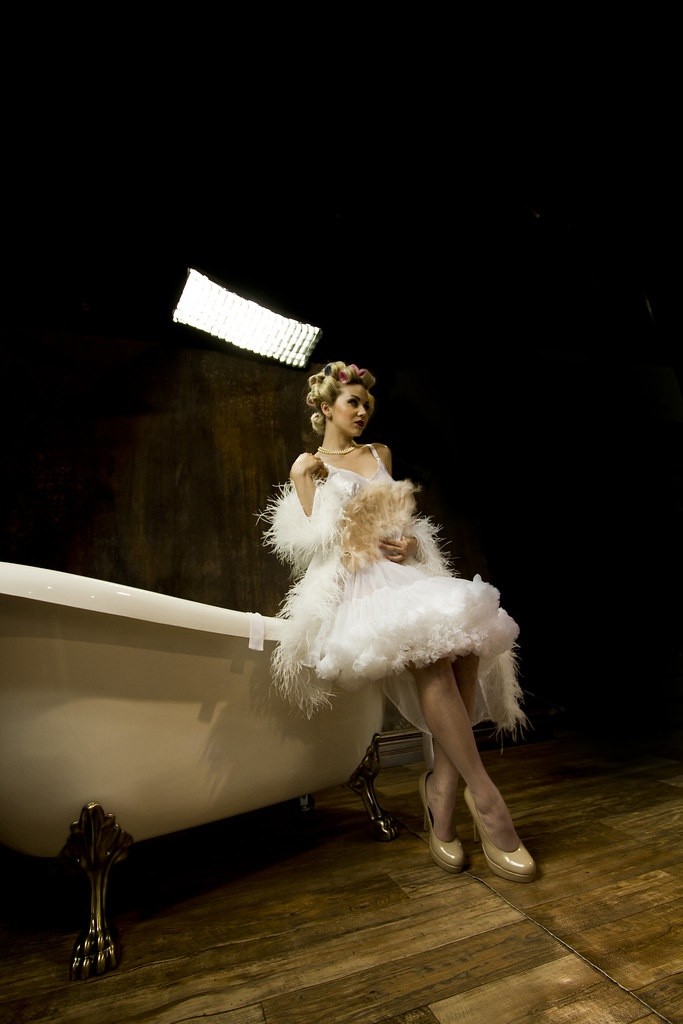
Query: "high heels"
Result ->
[[463, 786, 536, 883], [418, 769, 466, 874]]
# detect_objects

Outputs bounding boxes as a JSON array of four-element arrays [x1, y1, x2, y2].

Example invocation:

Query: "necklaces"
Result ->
[[317, 440, 357, 455]]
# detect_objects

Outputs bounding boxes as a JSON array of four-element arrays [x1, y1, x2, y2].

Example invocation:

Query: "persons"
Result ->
[[255, 355, 536, 883]]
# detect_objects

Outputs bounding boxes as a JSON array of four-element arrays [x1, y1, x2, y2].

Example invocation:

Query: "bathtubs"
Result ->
[[0, 560, 401, 983]]
